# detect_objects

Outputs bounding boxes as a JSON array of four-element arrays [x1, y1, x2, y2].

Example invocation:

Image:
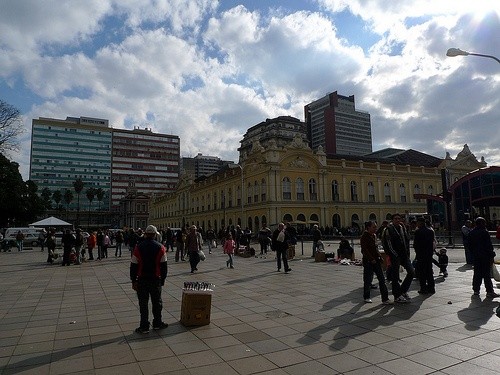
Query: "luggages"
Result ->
[[287, 244, 295, 259], [315, 242, 326, 262]]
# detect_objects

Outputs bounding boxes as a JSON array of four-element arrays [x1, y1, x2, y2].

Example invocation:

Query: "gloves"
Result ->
[[132, 280, 137, 290], [160, 276, 165, 286]]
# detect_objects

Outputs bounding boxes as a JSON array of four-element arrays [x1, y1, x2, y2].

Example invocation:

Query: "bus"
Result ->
[[398, 212, 433, 228], [2, 227, 47, 247]]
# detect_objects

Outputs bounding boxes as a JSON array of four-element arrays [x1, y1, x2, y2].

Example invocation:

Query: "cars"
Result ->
[[0, 233, 19, 251]]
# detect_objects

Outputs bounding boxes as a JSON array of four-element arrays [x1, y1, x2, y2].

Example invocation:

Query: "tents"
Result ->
[[29, 216, 73, 226]]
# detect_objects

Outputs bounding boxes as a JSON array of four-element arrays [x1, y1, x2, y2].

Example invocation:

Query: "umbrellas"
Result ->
[[291, 220, 320, 224]]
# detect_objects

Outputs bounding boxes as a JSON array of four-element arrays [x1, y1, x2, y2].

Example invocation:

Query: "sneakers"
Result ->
[[486, 291, 500, 298], [394, 295, 408, 303], [364, 298, 373, 303], [402, 294, 412, 301], [381, 299, 394, 304]]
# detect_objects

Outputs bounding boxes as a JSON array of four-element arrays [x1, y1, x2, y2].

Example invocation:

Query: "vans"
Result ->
[[169, 228, 181, 233], [54, 232, 63, 246]]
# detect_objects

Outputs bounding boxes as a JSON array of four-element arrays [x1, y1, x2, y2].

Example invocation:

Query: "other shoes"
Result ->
[[474, 289, 479, 295], [312, 256, 315, 258], [153, 322, 168, 329], [285, 268, 291, 272], [191, 268, 197, 273], [135, 326, 150, 333], [370, 285, 377, 289], [166, 250, 189, 262], [417, 287, 435, 294], [278, 268, 281, 272]]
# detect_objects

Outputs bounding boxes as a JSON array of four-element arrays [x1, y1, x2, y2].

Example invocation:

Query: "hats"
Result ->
[[465, 220, 472, 224], [417, 217, 425, 224], [313, 225, 318, 228], [287, 222, 291, 226], [146, 225, 160, 235], [382, 221, 388, 225], [191, 225, 197, 230]]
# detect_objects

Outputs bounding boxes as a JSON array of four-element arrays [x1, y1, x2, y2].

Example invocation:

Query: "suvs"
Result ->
[[81, 232, 90, 243]]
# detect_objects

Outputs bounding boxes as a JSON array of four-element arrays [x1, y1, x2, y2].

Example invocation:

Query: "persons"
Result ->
[[115, 226, 144, 258], [461, 217, 500, 298], [156, 224, 203, 273], [38, 227, 114, 266], [129, 226, 167, 334], [310, 224, 323, 258], [258, 222, 298, 260], [224, 236, 235, 269], [16, 231, 24, 251], [321, 224, 365, 236], [360, 213, 448, 304], [298, 225, 313, 235], [203, 225, 252, 255], [271, 223, 291, 272], [337, 237, 350, 258]]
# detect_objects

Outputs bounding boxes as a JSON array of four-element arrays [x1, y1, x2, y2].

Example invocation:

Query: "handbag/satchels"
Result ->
[[277, 230, 285, 242], [490, 262, 500, 282], [198, 249, 205, 261], [211, 239, 217, 248]]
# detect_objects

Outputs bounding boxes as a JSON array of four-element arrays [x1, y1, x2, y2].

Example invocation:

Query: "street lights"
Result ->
[[225, 161, 258, 231]]
